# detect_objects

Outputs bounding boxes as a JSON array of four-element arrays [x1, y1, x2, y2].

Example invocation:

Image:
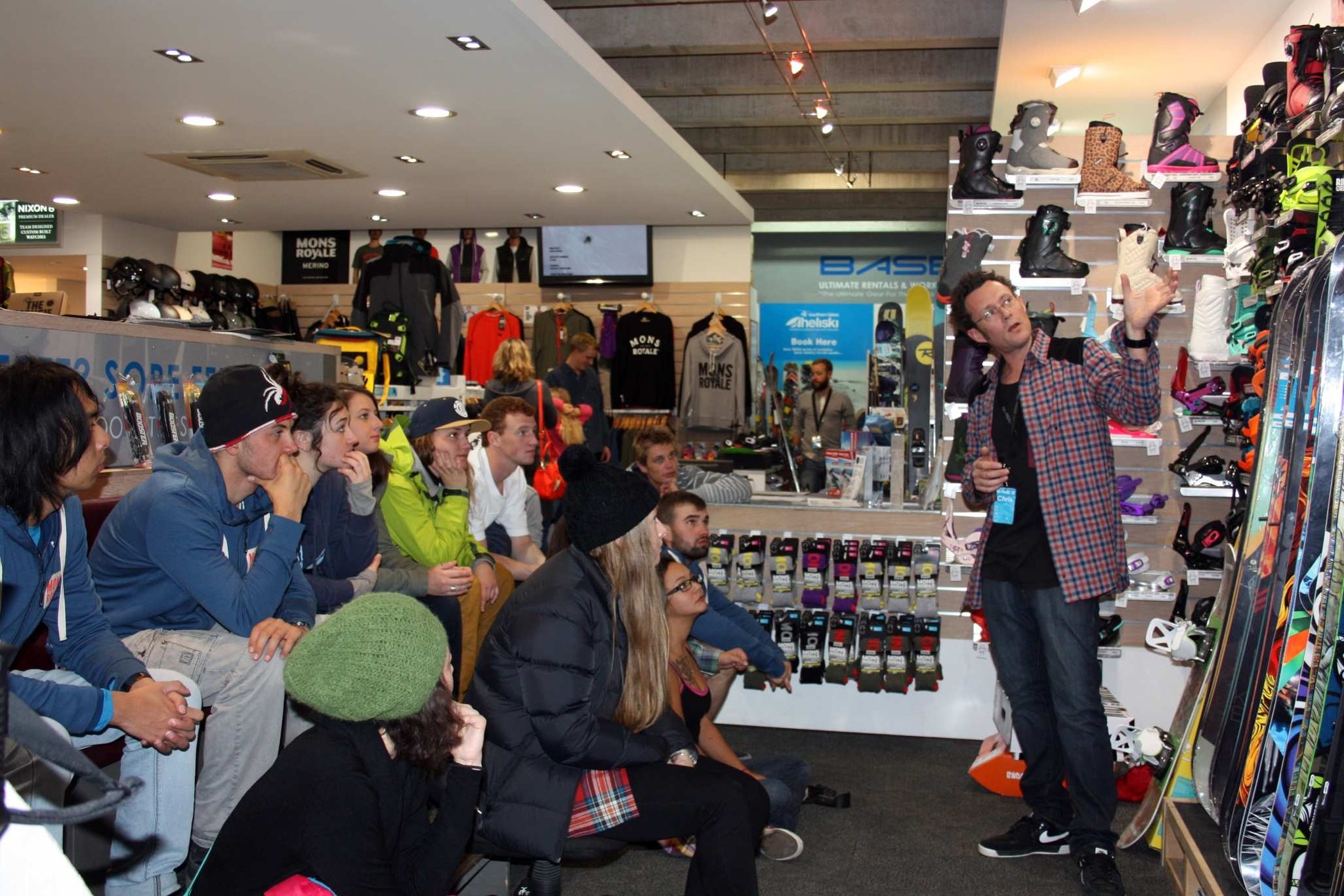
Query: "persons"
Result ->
[[648, 489, 795, 697], [325, 381, 475, 600], [3, 356, 205, 896], [490, 227, 539, 283], [410, 229, 439, 262], [482, 336, 564, 556], [444, 229, 489, 283], [792, 357, 858, 495], [630, 552, 807, 865], [621, 426, 753, 508], [182, 591, 504, 896], [352, 229, 386, 284], [87, 357, 336, 882], [368, 393, 516, 704], [457, 396, 552, 583], [267, 383, 385, 616], [538, 334, 612, 468], [948, 265, 1183, 895], [457, 441, 771, 896]]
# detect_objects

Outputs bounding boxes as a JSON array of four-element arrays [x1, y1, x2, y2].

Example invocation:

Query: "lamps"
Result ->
[[742, 0, 872, 194]]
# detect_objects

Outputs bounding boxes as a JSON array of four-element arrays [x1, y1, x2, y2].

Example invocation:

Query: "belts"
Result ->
[[1125, 236, 1344, 895]]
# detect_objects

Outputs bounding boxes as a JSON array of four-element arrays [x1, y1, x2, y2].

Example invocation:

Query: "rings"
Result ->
[[449, 585, 456, 593]]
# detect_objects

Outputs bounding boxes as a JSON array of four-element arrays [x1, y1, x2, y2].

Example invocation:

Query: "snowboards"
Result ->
[[1113, 543, 1240, 850]]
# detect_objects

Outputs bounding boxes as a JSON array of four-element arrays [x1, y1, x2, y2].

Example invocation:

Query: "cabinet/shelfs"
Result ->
[[0, 310, 342, 507], [962, 81, 1343, 666], [695, 500, 1005, 740]]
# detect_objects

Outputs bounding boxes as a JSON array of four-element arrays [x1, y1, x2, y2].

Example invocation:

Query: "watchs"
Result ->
[[293, 621, 312, 631], [669, 747, 700, 766]]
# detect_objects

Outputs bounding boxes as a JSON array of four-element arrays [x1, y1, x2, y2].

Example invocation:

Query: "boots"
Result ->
[[936, 227, 994, 303], [1241, 83, 1268, 148], [950, 124, 1024, 209], [1113, 225, 1183, 304], [1144, 90, 1224, 182], [1163, 184, 1226, 254], [1260, 60, 1293, 128], [943, 329, 985, 404], [943, 413, 966, 484], [1285, 24, 1324, 127], [1076, 121, 1152, 208], [1316, 27, 1344, 131], [1014, 203, 1090, 278], [1005, 99, 1083, 184]]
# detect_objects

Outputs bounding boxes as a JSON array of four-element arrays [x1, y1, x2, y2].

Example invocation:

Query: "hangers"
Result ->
[[484, 299, 729, 352]]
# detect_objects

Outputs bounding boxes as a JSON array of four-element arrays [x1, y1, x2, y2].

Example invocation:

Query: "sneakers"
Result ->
[[976, 812, 1070, 856], [1070, 834, 1125, 896], [734, 751, 752, 759], [758, 827, 802, 861]]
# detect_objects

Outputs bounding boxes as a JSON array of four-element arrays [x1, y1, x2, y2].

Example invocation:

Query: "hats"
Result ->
[[197, 364, 298, 452], [409, 397, 491, 437], [559, 444, 660, 550], [284, 592, 449, 722]]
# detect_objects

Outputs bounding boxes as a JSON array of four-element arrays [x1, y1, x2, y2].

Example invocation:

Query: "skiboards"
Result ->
[[1187, 234, 1343, 895]]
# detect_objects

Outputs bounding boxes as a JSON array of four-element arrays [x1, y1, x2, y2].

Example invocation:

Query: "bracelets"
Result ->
[[1121, 329, 1156, 349], [742, 767, 751, 774]]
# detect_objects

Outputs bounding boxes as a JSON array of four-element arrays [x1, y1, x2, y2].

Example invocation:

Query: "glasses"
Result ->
[[666, 574, 702, 597], [975, 295, 1015, 323]]
